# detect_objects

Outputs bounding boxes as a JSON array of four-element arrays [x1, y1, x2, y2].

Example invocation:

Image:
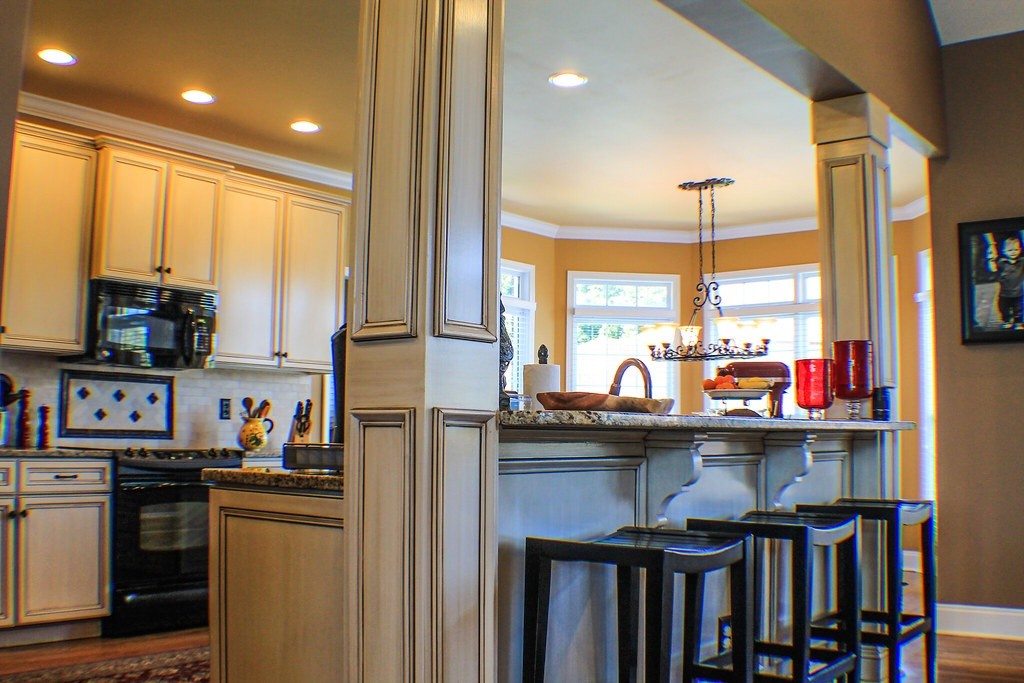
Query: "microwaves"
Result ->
[[60, 280, 219, 372]]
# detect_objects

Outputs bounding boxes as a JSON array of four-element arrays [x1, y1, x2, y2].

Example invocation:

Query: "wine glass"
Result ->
[[831, 340, 873, 420], [796, 359, 834, 420]]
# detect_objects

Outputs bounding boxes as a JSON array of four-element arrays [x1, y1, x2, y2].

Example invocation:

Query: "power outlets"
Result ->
[[220, 397, 231, 420]]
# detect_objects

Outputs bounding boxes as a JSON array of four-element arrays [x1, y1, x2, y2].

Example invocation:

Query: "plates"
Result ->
[[702, 389, 773, 398]]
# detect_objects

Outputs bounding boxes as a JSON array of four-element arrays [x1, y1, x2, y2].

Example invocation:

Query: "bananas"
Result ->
[[737, 377, 770, 389]]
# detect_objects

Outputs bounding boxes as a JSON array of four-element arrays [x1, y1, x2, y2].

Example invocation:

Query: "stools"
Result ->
[[681, 508, 864, 683], [795, 496, 936, 683], [523, 524, 755, 683]]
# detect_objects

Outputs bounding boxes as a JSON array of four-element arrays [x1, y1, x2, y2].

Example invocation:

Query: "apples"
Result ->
[[703, 375, 737, 389]]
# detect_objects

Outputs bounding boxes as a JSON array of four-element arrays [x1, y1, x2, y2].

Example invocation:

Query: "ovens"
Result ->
[[103, 479, 212, 637]]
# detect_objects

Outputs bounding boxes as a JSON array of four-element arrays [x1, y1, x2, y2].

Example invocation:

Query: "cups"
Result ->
[[238, 416, 274, 451]]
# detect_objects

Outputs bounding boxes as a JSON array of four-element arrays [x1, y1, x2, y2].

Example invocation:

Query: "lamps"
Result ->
[[642, 177, 774, 360]]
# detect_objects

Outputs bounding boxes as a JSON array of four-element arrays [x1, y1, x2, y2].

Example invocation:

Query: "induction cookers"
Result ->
[[56, 446, 242, 478]]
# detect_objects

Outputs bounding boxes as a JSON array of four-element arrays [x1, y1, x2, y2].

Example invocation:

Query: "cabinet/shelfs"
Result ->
[[0, 452, 112, 642], [0, 117, 353, 375]]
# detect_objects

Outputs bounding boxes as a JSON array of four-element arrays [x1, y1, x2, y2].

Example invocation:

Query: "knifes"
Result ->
[[295, 399, 312, 422]]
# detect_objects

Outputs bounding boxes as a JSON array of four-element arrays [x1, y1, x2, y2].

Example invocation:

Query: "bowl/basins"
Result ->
[[660, 399, 675, 413], [620, 398, 663, 412], [594, 395, 618, 409], [535, 391, 609, 409], [508, 393, 532, 410]]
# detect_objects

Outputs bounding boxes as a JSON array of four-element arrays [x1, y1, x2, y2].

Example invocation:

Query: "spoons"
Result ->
[[242, 397, 254, 417]]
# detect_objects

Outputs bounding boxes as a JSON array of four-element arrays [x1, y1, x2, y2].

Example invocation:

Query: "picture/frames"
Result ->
[[957, 216, 1024, 344]]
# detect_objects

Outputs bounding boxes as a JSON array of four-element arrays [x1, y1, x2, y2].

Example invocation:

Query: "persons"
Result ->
[[994, 236, 1024, 330]]
